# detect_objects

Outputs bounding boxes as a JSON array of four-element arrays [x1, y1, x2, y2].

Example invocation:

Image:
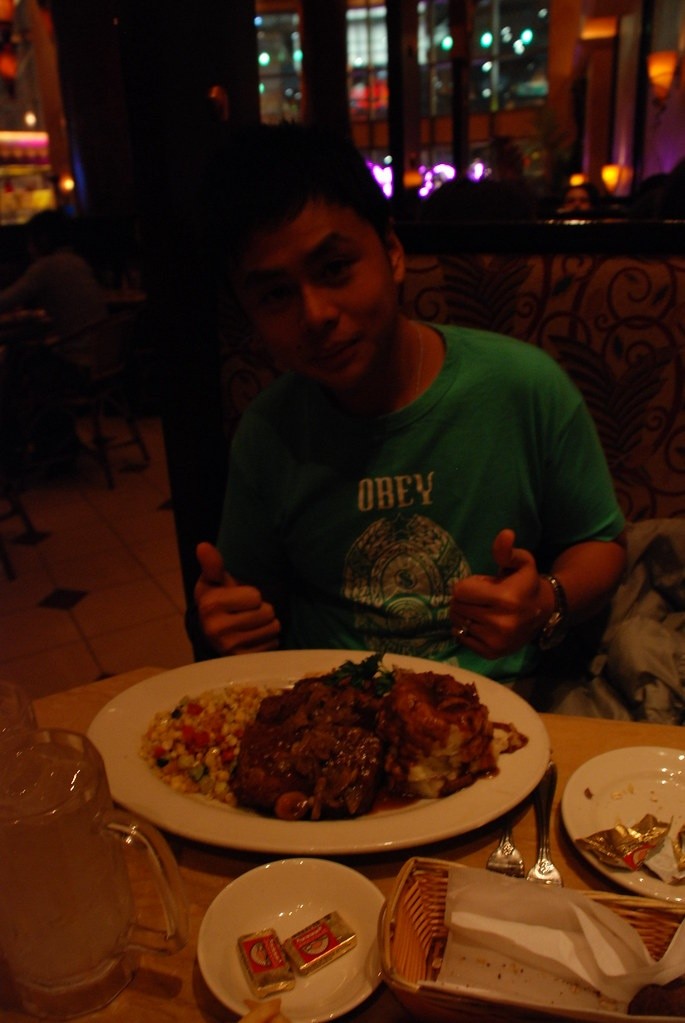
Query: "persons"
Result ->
[[421, 137, 537, 220], [621, 160, 685, 254], [0, 212, 118, 477], [192, 124, 629, 722]]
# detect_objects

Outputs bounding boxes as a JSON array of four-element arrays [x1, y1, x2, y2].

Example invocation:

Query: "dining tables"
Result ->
[[0, 665, 685, 1023]]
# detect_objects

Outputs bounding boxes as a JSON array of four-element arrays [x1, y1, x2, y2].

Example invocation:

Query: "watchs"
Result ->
[[532, 575, 569, 650]]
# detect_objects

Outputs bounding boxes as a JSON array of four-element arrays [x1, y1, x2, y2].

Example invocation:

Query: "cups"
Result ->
[[0, 684, 188, 1021]]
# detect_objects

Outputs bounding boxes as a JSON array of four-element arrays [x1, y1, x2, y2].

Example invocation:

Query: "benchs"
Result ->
[[144, 224, 685, 669]]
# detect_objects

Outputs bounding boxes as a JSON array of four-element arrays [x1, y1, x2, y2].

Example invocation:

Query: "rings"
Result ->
[[455, 626, 468, 644]]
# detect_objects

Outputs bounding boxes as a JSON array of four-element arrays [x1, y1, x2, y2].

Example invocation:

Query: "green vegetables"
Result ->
[[320, 645, 397, 700]]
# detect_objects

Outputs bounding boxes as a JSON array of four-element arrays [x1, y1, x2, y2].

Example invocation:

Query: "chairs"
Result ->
[[40, 312, 153, 487]]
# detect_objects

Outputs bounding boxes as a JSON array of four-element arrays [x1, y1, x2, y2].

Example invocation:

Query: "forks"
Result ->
[[488, 758, 563, 888]]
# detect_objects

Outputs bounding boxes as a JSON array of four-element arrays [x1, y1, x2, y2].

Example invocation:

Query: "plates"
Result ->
[[196, 859, 388, 1023], [86, 649, 550, 856], [560, 746, 685, 908]]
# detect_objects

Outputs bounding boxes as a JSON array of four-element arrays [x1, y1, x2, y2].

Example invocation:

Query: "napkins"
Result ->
[[433, 864, 685, 1013]]
